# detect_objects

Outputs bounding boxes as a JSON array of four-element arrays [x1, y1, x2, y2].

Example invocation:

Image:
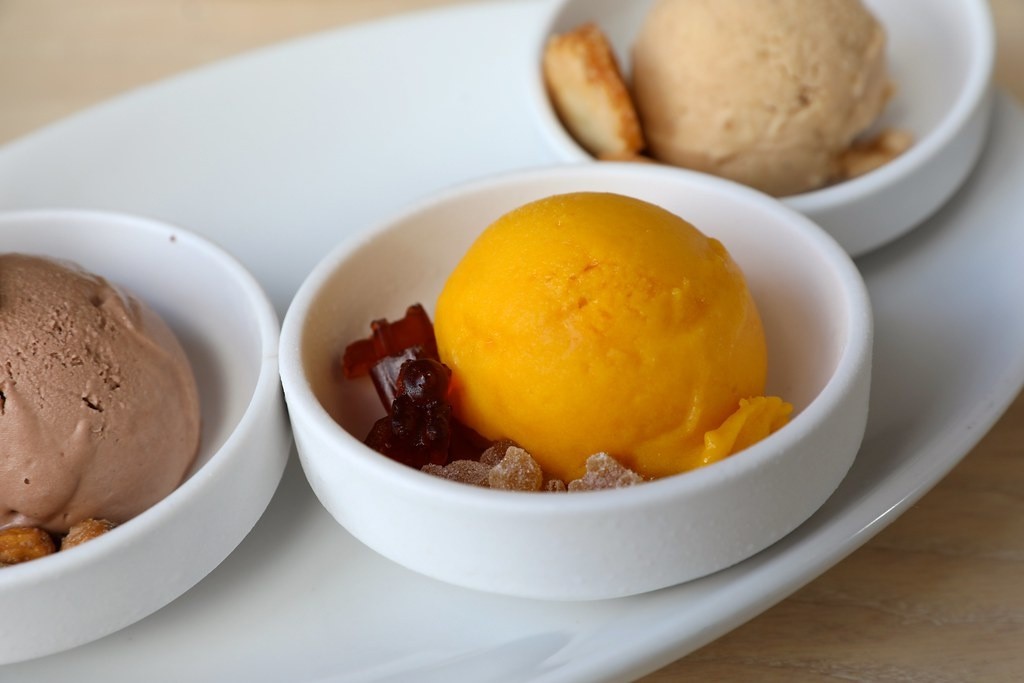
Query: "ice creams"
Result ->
[[0, 249, 201, 566], [338, 190, 793, 497], [542, 0, 912, 195]]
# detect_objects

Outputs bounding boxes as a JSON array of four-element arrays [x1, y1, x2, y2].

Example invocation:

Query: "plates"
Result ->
[[518, 0, 1001, 258], [280, 160, 878, 607], [0, 207, 292, 663], [0, 0, 1024, 683]]
